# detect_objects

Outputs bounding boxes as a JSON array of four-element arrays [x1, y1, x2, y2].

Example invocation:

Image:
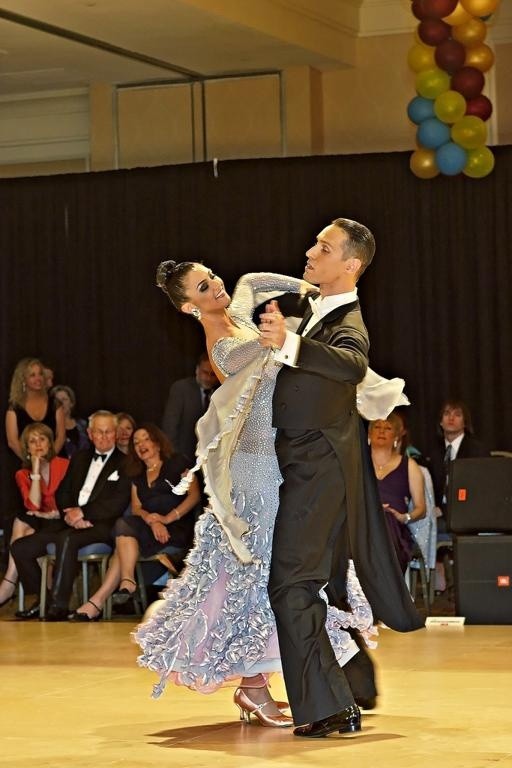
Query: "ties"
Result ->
[[203, 389, 211, 414], [443, 444, 453, 484]]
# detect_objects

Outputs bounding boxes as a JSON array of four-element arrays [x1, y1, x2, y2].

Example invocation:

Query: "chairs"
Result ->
[[1, 447, 198, 623], [397, 462, 443, 615], [422, 522, 456, 613]]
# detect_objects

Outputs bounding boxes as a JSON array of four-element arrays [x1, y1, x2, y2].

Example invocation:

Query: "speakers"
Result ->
[[449, 457, 512, 533], [453, 536, 512, 625]]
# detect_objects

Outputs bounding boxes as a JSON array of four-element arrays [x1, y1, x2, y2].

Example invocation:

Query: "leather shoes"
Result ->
[[119, 578, 137, 594], [38, 608, 70, 621], [292, 705, 362, 736], [69, 600, 104, 621], [15, 599, 43, 617], [356, 696, 376, 710]]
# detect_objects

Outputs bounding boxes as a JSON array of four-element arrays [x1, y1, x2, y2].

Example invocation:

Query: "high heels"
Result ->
[[237, 689, 294, 727], [234, 688, 290, 720], [0, 578, 17, 609]]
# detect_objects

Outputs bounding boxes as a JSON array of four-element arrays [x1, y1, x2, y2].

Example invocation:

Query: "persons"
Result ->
[[0, 358, 219, 624], [423, 399, 488, 600], [128, 258, 322, 730], [365, 416, 428, 577], [255, 215, 426, 741]]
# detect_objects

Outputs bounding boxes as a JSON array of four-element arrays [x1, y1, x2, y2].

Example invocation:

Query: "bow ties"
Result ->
[[91, 452, 108, 462]]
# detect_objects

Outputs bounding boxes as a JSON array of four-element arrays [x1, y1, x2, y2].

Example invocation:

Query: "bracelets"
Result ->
[[403, 513, 412, 524]]
